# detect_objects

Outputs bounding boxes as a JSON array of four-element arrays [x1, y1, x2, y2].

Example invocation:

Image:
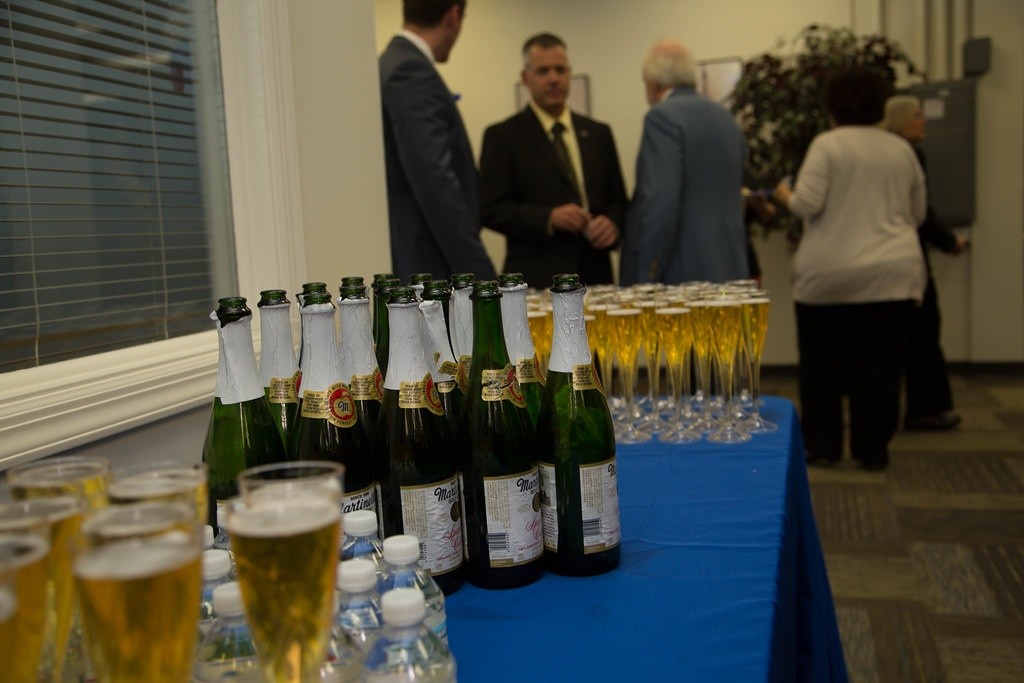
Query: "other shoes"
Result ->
[[860, 457, 889, 471], [805, 451, 844, 468], [904, 412, 960, 431]]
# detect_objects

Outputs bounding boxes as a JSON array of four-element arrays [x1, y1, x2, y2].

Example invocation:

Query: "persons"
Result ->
[[773, 67, 934, 473], [878, 95, 967, 432], [378, 0, 501, 280], [617, 41, 766, 290], [742, 161, 776, 288], [479, 30, 629, 288], [745, 138, 787, 233]]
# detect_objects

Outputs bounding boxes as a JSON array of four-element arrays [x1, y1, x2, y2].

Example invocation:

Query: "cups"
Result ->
[[238, 463, 346, 501], [218, 496, 343, 683], [0, 462, 207, 683]]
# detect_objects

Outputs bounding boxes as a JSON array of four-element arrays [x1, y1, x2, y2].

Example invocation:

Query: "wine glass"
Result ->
[[528, 280, 777, 444]]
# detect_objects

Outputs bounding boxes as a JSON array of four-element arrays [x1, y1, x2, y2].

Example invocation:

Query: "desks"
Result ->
[[201, 389, 850, 683]]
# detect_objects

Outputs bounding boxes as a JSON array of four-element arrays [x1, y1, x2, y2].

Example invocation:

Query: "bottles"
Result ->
[[199, 276, 622, 683]]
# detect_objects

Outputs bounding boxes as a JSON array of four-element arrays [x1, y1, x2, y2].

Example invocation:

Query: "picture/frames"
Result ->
[[516, 75, 590, 117], [699, 56, 746, 111]]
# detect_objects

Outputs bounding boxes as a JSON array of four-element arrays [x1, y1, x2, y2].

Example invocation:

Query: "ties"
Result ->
[[551, 123, 584, 206]]
